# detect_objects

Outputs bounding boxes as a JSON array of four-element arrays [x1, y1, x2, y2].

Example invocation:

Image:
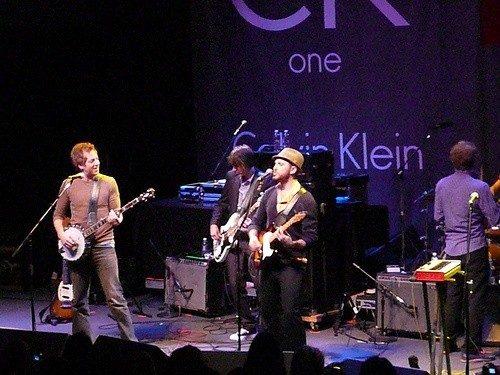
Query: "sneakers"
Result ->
[[230, 327, 257, 341], [441, 346, 457, 354], [461, 349, 495, 362]]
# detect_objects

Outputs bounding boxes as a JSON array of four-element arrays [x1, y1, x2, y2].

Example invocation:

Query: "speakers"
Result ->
[[164, 256, 230, 315], [375, 271, 438, 335], [0, 329, 70, 358], [339, 359, 431, 375], [95, 335, 170, 364], [201, 351, 293, 375]]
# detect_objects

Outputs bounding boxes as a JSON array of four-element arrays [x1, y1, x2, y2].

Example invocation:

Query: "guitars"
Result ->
[[57, 187, 157, 262], [253, 210, 309, 269], [213, 177, 266, 264], [50, 257, 76, 321]]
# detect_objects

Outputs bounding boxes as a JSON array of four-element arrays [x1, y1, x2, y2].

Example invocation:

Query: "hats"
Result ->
[[272, 148, 304, 176]]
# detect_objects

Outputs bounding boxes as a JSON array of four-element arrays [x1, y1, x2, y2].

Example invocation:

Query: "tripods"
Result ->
[[369, 129, 438, 273]]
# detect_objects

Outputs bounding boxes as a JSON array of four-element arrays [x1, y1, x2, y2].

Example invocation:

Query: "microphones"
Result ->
[[234, 120, 247, 135], [68, 172, 85, 179], [175, 289, 193, 292], [407, 304, 417, 310], [431, 120, 454, 129], [256, 168, 273, 181], [469, 192, 479, 204]]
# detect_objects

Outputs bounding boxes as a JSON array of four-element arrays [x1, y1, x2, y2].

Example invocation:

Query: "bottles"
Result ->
[[336, 197, 350, 202], [431, 252, 438, 261], [201, 238, 209, 258]]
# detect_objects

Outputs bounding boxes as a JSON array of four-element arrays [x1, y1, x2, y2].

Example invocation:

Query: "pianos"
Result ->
[[412, 257, 462, 375]]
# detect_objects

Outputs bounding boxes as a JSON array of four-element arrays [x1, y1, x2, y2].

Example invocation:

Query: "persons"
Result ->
[[434, 140, 500, 362], [359, 356, 396, 375], [210, 144, 270, 340], [0, 329, 325, 375], [53, 142, 138, 342], [248, 148, 318, 351]]
